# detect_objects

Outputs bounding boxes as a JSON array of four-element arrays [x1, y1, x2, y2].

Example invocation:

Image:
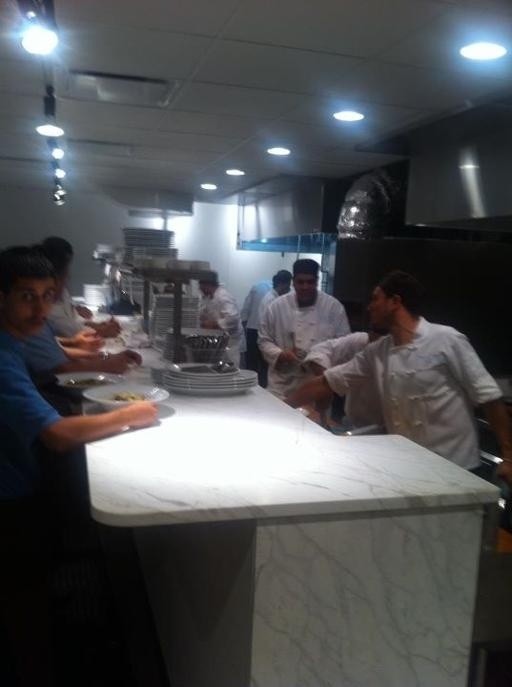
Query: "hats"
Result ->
[[293, 260, 319, 274], [380, 271, 427, 310]]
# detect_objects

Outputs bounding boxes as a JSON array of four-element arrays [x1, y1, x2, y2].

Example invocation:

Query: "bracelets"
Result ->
[[502, 455, 512, 465]]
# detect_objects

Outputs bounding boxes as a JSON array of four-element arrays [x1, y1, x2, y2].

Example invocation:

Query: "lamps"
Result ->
[[13, 1, 70, 208]]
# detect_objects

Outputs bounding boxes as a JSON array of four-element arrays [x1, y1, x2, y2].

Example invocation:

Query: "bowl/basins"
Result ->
[[220, 308, 240, 332]]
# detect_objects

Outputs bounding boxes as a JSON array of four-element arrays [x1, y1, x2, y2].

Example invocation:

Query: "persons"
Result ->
[[0, 243, 161, 506], [194, 268, 250, 372], [283, 267, 511, 484], [0, 233, 144, 377], [241, 280, 270, 367], [257, 257, 352, 402], [256, 270, 293, 344], [302, 325, 389, 435]]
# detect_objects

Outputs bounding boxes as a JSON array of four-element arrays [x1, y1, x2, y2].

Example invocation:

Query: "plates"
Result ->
[[125, 228, 230, 358], [82, 384, 167, 406], [57, 372, 127, 388], [152, 361, 258, 393], [83, 283, 113, 305]]
